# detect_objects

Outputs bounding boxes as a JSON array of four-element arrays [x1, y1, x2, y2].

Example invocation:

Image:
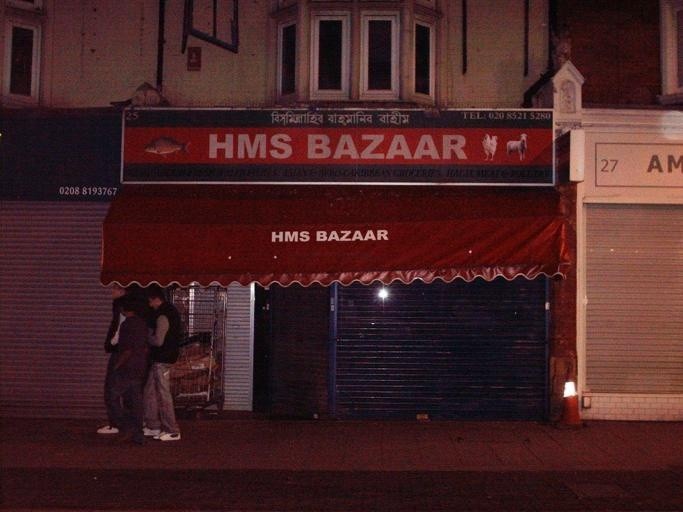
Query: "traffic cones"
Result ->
[[554, 360, 589, 430]]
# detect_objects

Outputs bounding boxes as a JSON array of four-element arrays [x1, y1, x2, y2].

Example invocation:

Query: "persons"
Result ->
[[95, 295, 135, 433], [142, 287, 181, 442], [109, 299, 150, 444]]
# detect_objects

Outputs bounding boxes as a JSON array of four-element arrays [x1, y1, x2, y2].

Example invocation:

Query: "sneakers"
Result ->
[[160, 432, 181, 441], [141, 425, 161, 436], [96, 425, 119, 434], [153, 431, 169, 440]]
